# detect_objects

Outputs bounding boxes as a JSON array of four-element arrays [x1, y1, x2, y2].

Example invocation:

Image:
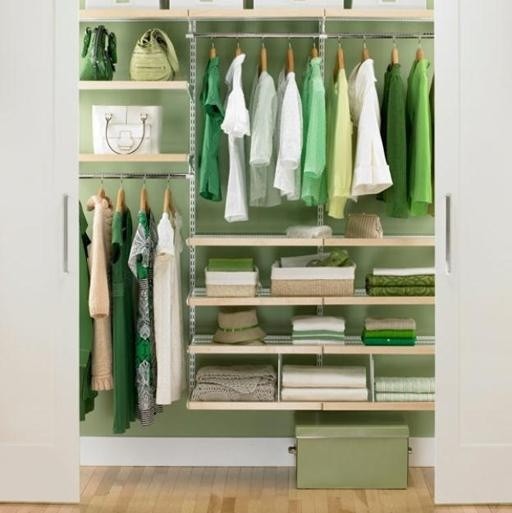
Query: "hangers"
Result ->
[[208, 33, 426, 76], [97, 173, 172, 214]]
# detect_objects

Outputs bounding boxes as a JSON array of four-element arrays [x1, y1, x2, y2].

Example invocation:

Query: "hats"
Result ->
[[213, 308, 265, 343]]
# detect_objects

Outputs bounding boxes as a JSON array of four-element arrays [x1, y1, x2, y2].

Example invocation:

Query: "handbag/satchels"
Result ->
[[130, 27, 179, 80], [92, 106, 162, 155], [344, 213, 382, 239], [79, 25, 116, 80]]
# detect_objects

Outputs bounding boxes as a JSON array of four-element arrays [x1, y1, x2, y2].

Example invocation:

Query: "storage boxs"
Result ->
[[290, 416, 410, 489]]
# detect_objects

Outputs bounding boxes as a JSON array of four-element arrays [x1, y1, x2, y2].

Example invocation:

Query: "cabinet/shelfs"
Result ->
[[0, 0, 511, 504]]
